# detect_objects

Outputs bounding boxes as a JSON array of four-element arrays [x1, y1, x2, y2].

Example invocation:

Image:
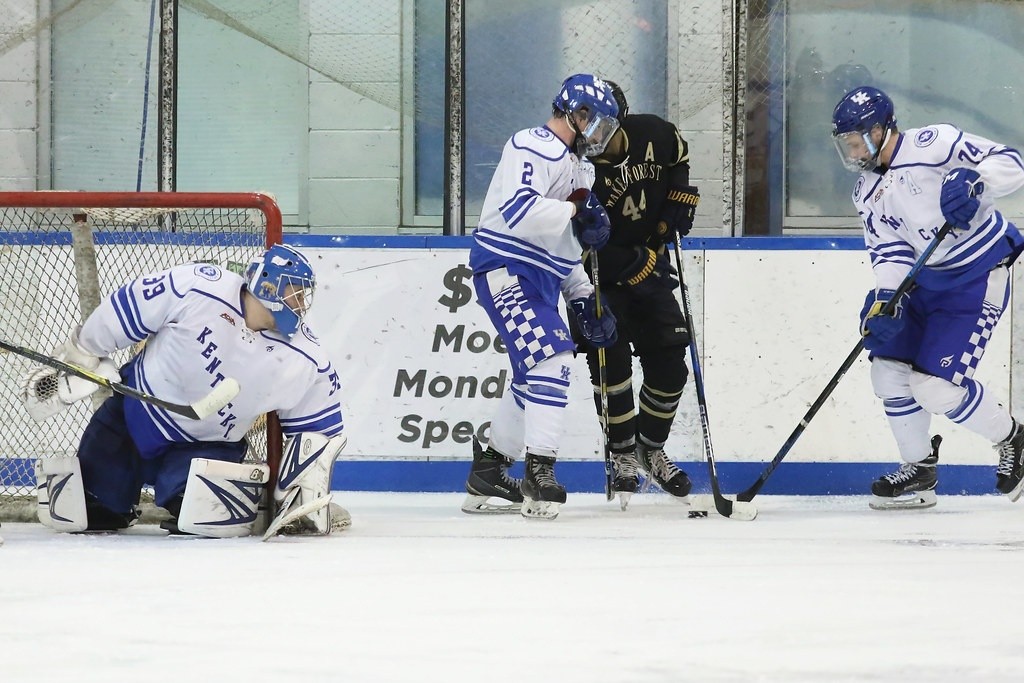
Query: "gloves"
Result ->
[[568, 188, 609, 251], [614, 244, 679, 301], [941, 169, 983, 230], [651, 185, 701, 245], [859, 288, 910, 349], [570, 290, 617, 348]]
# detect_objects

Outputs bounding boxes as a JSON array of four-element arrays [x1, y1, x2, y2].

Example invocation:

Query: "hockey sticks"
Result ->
[[0, 338, 240, 420], [262, 487, 333, 541], [590, 246, 613, 502], [672, 226, 758, 522], [721, 222, 952, 502]]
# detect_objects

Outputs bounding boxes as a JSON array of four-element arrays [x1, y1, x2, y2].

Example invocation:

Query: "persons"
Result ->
[[831, 86, 1024, 509], [20, 244, 351, 540], [568, 82, 701, 510], [460, 71, 619, 518]]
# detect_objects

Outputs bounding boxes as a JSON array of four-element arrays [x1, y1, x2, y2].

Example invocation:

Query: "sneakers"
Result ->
[[461, 435, 525, 514], [634, 441, 692, 506], [868, 434, 941, 509], [611, 453, 641, 509], [518, 446, 566, 520], [992, 417, 1024, 502]]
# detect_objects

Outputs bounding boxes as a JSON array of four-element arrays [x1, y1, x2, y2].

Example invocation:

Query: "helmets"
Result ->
[[832, 87, 894, 171], [246, 244, 316, 342], [603, 79, 629, 124], [552, 73, 618, 157]]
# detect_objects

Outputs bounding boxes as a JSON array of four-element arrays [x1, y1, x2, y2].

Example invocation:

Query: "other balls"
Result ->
[[689, 510, 708, 519]]
